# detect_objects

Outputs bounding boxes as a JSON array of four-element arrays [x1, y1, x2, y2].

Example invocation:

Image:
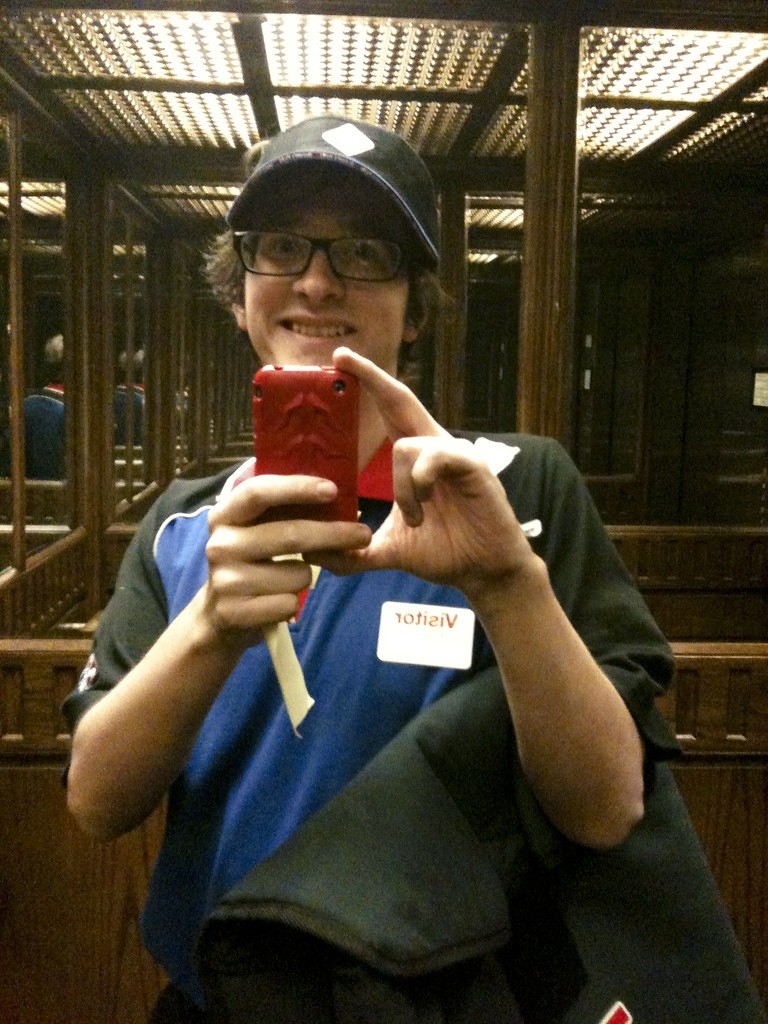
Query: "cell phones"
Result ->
[[253, 364, 359, 522]]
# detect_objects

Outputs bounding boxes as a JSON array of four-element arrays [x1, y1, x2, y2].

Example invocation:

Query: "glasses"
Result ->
[[233, 231, 411, 282]]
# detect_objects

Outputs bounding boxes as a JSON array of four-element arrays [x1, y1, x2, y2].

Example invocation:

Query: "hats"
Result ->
[[226, 115, 441, 274]]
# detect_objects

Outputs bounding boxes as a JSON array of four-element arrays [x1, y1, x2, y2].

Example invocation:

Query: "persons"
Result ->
[[69, 113, 768, 1024], [134, 349, 145, 446], [113, 351, 127, 447], [0, 334, 66, 480]]
